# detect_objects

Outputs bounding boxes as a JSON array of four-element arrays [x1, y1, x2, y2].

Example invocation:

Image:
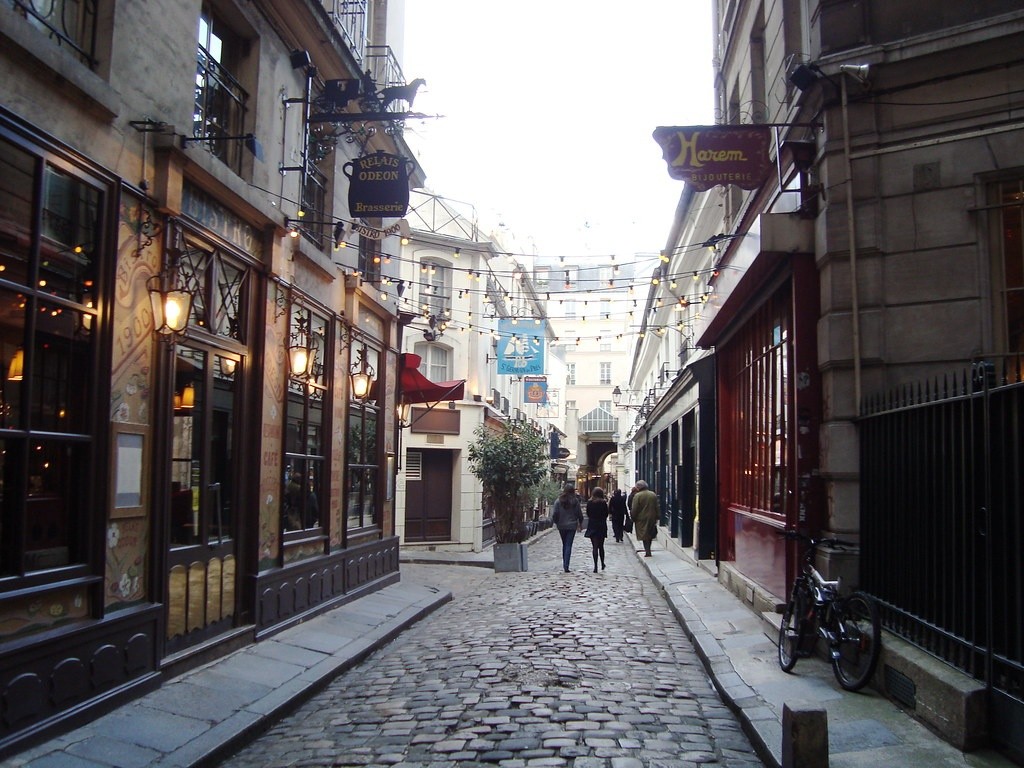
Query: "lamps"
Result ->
[[274, 280, 320, 381], [359, 279, 406, 297], [181, 129, 266, 167], [510, 374, 523, 385], [298, 206, 309, 217], [612, 433, 631, 451], [284, 216, 346, 245], [626, 368, 682, 437], [339, 320, 375, 400], [611, 386, 642, 412], [486, 397, 493, 404], [290, 49, 312, 71], [473, 395, 482, 401], [397, 309, 437, 329], [403, 325, 437, 342], [324, 79, 361, 106], [137, 202, 195, 346], [840, 64, 869, 80]]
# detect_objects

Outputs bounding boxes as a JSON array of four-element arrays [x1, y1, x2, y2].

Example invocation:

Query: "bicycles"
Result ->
[[775, 530, 882, 692]]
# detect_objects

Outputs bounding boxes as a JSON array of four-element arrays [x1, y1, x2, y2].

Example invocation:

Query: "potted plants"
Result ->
[[466, 417, 555, 572], [539, 477, 561, 519]]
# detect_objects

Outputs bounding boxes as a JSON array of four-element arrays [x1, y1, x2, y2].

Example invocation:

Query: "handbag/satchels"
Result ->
[[624, 513, 633, 532], [651, 526, 657, 537]]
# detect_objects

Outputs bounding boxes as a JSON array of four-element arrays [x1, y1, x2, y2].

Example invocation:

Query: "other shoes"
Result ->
[[602, 564, 605, 569], [564, 566, 570, 572], [593, 570, 597, 573], [616, 539, 619, 542], [644, 554, 652, 557], [621, 538, 623, 541]]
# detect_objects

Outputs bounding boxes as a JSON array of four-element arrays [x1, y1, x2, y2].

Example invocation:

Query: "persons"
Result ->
[[584, 487, 609, 573], [553, 483, 583, 572], [631, 479, 658, 558], [609, 487, 638, 542]]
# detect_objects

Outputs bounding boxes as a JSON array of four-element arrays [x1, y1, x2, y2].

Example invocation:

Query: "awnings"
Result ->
[[398, 353, 464, 405]]
